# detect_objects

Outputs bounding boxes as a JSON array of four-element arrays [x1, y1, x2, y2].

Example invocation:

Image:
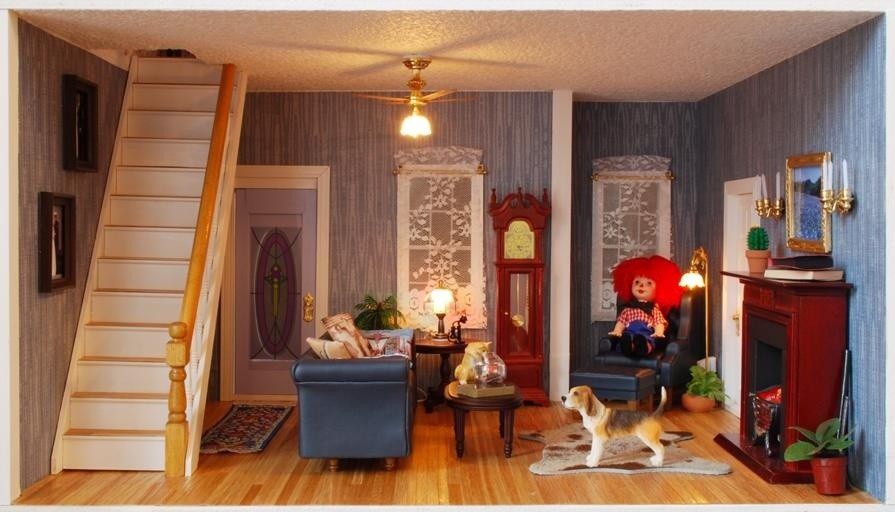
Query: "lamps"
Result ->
[[679, 246, 712, 371], [429, 289, 455, 338], [400, 107, 432, 137]]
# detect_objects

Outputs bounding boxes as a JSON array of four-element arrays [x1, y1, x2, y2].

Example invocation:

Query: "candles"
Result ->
[[756, 159, 849, 200]]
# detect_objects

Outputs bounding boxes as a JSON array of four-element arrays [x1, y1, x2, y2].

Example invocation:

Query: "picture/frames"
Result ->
[[783, 150, 834, 252], [38, 75, 98, 292]]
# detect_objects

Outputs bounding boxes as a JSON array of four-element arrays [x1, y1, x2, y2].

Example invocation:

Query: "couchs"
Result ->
[[290, 328, 416, 472]]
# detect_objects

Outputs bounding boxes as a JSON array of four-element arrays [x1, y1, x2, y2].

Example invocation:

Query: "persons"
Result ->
[[607, 255, 689, 356]]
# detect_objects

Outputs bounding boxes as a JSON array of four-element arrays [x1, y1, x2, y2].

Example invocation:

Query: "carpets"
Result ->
[[519, 421, 733, 476], [200, 404, 294, 455]]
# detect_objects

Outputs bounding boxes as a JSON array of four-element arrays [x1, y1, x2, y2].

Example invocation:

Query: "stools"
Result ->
[[571, 365, 655, 414]]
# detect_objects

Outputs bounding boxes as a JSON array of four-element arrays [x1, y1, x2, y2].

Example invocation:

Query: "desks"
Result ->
[[415, 338, 480, 413]]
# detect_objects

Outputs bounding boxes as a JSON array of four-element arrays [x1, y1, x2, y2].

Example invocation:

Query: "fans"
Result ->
[[348, 58, 477, 106]]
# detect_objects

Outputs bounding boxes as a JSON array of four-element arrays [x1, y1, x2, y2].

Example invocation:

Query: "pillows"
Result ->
[[359, 329, 414, 360], [305, 337, 355, 360], [321, 312, 371, 357]]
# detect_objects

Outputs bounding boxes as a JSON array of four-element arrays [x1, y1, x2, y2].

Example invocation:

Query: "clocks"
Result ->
[[488, 189, 552, 404]]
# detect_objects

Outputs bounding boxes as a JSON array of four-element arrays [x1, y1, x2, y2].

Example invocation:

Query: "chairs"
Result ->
[[600, 289, 695, 411]]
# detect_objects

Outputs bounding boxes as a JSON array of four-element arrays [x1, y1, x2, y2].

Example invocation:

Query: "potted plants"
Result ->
[[681, 365, 730, 412], [746, 227, 771, 272], [783, 418, 860, 496]]
